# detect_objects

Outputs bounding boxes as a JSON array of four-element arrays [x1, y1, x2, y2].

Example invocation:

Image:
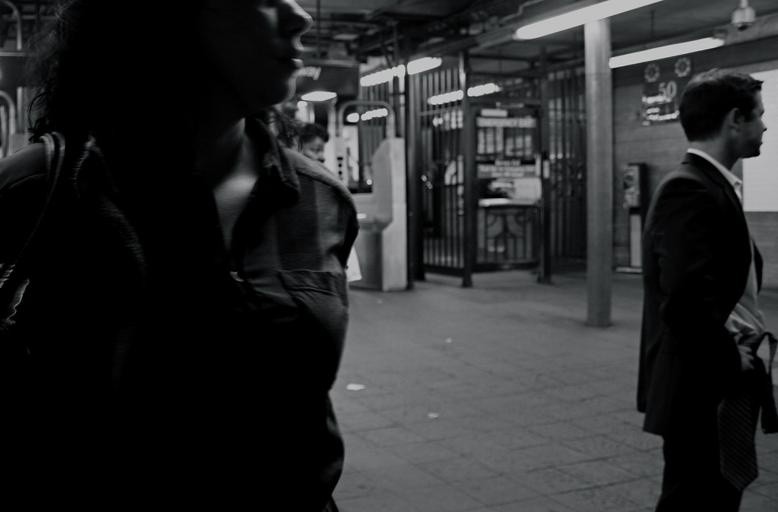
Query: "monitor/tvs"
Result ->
[[474, 159, 545, 209], [478, 103, 544, 162]]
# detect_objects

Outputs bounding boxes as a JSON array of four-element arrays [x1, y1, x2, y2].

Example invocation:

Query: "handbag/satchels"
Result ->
[[1, 128, 70, 340]]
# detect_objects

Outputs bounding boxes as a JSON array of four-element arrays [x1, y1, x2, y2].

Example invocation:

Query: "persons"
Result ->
[[298, 122, 363, 284], [637, 67, 778, 512], [1, 1, 361, 512]]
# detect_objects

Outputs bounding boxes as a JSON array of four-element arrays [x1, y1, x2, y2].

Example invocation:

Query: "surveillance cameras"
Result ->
[[729, 8, 756, 31]]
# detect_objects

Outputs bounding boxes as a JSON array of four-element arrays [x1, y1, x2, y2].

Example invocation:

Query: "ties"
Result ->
[[717, 331, 777, 493]]
